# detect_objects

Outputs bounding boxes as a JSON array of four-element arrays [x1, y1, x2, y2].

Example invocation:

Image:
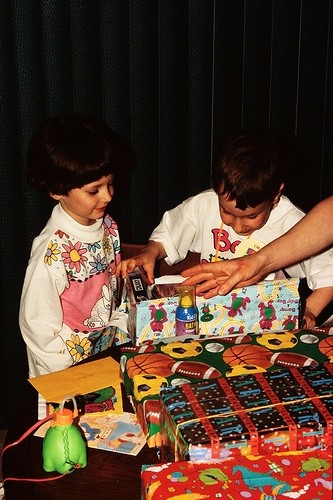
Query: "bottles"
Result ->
[[42, 396, 88, 475], [175, 285, 199, 336]]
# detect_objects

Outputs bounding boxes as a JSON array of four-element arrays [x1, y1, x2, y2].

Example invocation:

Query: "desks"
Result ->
[[0, 417, 157, 500]]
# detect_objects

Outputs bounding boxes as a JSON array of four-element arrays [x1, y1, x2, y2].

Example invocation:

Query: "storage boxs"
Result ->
[[123, 270, 300, 347], [119, 329, 333, 461], [141, 445, 333, 500]]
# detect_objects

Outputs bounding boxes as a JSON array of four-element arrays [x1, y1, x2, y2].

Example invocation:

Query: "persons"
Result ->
[[180, 195, 333, 300], [113, 139, 333, 327], [17, 118, 121, 421]]
